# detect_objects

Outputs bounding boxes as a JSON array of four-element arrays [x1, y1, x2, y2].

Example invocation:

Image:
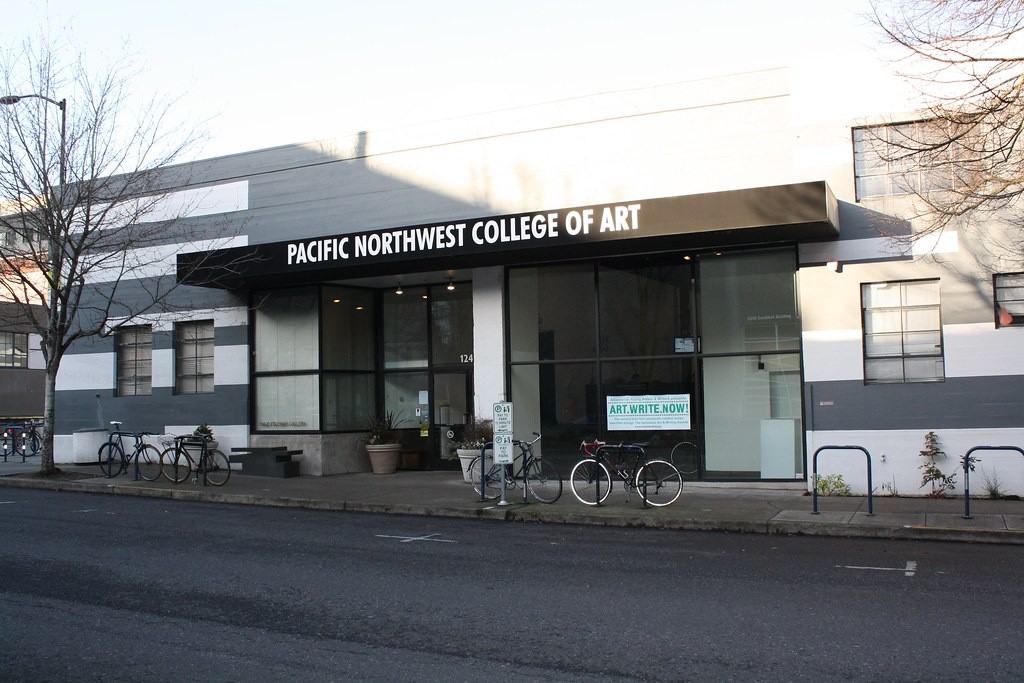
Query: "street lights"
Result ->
[[0, 92, 68, 237]]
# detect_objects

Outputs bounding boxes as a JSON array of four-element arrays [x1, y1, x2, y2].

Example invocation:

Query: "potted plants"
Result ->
[[183, 421, 219, 471]]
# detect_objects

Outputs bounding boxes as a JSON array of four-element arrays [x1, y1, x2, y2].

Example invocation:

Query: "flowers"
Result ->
[[445, 416, 494, 462], [354, 408, 414, 450]]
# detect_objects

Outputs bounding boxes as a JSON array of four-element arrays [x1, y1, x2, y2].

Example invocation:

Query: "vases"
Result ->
[[456, 449, 496, 485], [366, 444, 402, 475]]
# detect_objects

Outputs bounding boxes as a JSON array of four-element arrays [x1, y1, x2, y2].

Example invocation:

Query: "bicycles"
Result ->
[[569, 438, 684, 508], [156, 432, 231, 487], [97, 420, 163, 482], [465, 431, 563, 504], [670, 441, 705, 474], [0, 418, 45, 457]]
